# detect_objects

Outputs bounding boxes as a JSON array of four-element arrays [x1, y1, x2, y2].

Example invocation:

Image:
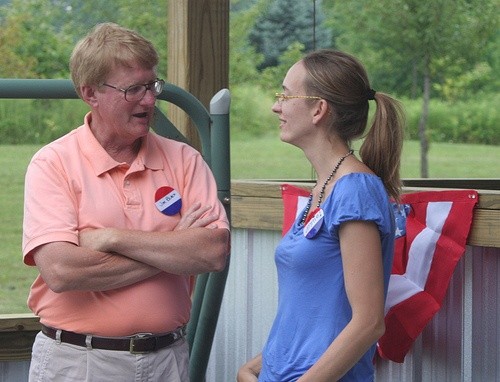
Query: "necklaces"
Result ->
[[300, 150, 353, 225]]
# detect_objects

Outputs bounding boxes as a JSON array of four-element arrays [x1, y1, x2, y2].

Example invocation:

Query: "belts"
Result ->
[[39, 322, 188, 355]]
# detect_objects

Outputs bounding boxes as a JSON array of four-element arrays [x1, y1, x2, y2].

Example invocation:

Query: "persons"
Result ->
[[21, 21, 232, 381], [236, 50, 396, 382]]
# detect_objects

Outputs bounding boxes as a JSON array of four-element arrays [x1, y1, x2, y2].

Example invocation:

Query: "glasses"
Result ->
[[274, 92, 324, 104], [97, 78, 165, 103]]
